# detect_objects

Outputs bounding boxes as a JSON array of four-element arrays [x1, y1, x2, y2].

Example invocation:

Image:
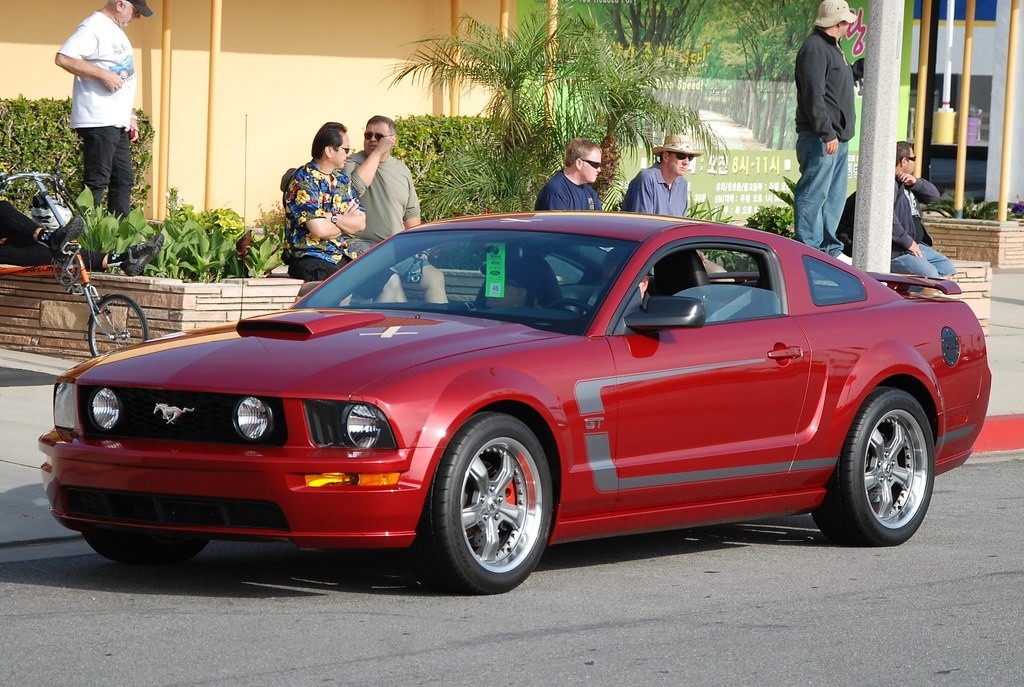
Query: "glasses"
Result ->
[[901, 156, 916, 162], [582, 159, 601, 169], [338, 146, 350, 154], [363, 131, 394, 142], [666, 151, 695, 161]]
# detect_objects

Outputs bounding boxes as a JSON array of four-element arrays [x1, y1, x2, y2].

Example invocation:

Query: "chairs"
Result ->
[[654, 248, 707, 295], [513, 254, 566, 310]]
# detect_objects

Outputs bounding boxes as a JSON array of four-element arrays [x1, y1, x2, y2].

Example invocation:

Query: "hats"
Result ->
[[127, 0, 153, 17], [652, 135, 704, 157], [814, 0, 858, 27], [480, 260, 530, 287]]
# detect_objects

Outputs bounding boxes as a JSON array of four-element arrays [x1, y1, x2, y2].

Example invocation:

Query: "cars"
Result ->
[[37, 210, 991, 595]]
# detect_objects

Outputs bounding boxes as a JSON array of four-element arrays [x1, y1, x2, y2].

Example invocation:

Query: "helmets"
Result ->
[[33, 195, 72, 232]]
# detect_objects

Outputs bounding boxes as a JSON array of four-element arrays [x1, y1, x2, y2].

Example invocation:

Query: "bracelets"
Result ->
[[131, 114, 138, 120]]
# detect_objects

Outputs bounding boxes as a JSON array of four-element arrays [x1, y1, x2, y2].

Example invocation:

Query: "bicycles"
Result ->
[[0, 165, 149, 359]]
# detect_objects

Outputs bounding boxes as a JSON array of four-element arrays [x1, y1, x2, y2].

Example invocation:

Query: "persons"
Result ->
[[604, 249, 654, 311], [480, 256, 536, 314], [534, 137, 603, 211], [283, 122, 408, 305], [890, 141, 958, 294], [55, 0, 154, 222], [795, 0, 857, 265], [0, 200, 164, 276], [620, 134, 735, 282], [341, 115, 448, 304]]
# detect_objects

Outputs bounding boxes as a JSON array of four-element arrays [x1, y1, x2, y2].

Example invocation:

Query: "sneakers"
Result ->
[[119, 234, 164, 277], [48, 215, 84, 262]]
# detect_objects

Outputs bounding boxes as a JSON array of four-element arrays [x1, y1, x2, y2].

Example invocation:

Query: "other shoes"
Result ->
[[835, 252, 853, 266]]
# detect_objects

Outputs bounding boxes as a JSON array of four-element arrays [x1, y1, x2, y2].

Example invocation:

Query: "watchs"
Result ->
[[331, 214, 338, 225]]
